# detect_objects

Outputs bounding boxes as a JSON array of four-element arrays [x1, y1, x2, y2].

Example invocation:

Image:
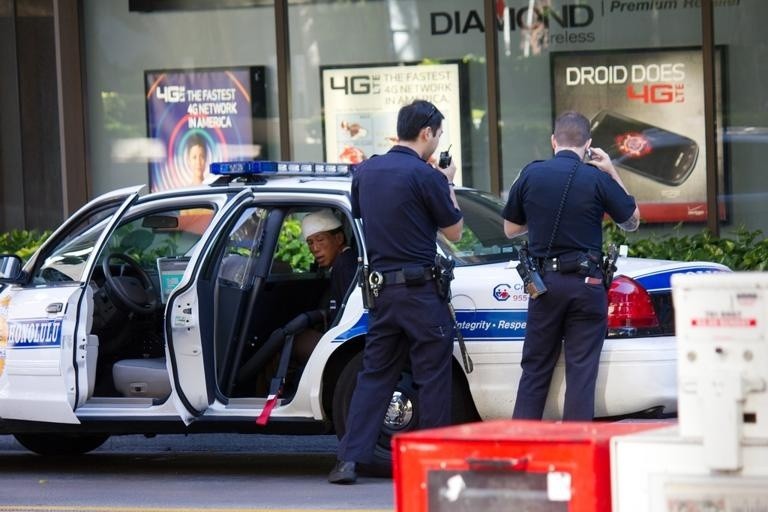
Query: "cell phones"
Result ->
[[590, 107, 700, 187]]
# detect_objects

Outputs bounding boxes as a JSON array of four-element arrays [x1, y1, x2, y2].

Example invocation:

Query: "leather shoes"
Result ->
[[326, 458, 358, 485]]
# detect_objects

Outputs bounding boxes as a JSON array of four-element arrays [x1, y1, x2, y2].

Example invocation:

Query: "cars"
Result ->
[[0, 161, 732, 480]]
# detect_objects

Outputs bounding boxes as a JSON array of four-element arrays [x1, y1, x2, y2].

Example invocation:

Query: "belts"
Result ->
[[382, 264, 439, 288], [539, 253, 603, 273]]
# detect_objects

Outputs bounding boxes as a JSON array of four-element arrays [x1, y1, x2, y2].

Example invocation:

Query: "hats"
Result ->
[[301, 206, 345, 242]]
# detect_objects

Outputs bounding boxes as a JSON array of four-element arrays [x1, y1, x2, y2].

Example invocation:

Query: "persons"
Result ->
[[182, 135, 210, 187], [324, 99, 466, 490], [502, 110, 641, 422], [230, 206, 360, 401]]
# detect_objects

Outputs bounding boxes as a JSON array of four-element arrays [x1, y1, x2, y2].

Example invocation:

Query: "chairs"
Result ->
[[110, 214, 292, 398]]
